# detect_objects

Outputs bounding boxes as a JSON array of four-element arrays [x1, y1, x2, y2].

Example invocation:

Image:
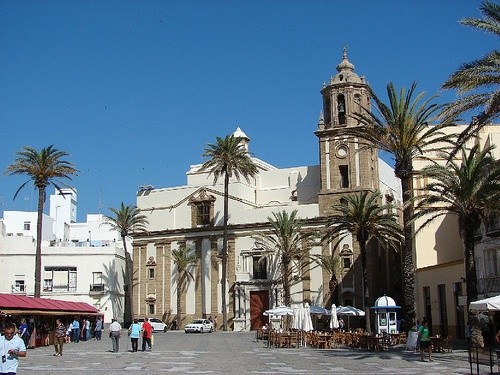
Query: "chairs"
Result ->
[[256, 328, 455, 355]]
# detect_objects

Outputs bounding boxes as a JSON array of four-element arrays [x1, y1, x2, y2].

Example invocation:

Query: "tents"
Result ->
[[468, 296, 500, 375]]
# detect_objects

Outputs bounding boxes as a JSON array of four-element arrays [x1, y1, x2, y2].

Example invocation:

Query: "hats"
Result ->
[[114, 317, 117, 321]]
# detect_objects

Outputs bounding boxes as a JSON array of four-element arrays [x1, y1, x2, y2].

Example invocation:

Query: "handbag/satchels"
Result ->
[[128, 329, 131, 336]]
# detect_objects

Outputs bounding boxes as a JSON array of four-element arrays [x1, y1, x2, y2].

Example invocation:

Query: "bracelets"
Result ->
[[16, 351, 18, 355]]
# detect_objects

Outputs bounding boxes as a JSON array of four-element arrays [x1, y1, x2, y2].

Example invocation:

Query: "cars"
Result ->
[[137, 318, 168, 333], [185, 319, 215, 333]]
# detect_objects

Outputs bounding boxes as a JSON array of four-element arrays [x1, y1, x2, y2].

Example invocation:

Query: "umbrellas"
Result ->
[[292, 302, 314, 349], [263, 302, 365, 332]]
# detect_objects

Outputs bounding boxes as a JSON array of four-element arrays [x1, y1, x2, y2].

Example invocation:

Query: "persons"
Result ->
[[0, 322, 26, 375], [339, 316, 345, 328], [68, 316, 102, 343], [417, 316, 434, 362], [54, 318, 66, 357], [0, 318, 50, 349], [208, 315, 218, 332], [173, 319, 177, 330], [128, 319, 141, 352], [141, 318, 153, 351], [109, 318, 122, 352]]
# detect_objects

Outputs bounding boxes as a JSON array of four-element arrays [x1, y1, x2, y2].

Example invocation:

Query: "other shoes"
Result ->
[[77, 341, 79, 343], [56, 353, 59, 356], [58, 354, 62, 357]]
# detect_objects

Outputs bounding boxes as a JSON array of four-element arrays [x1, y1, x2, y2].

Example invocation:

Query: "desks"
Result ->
[[319, 335, 334, 348], [389, 333, 406, 344], [366, 337, 385, 350], [430, 337, 442, 352]]
